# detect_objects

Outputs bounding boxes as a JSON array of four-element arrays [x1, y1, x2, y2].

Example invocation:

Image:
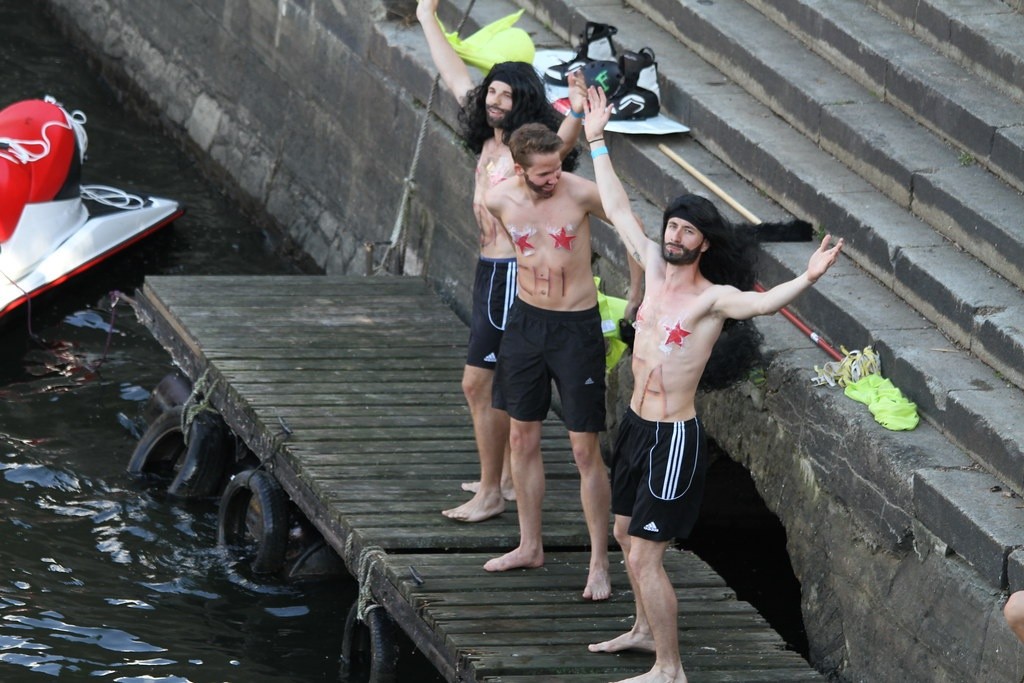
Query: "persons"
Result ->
[[414, 0, 588, 523], [484, 124, 648, 601], [582, 84, 843, 683]]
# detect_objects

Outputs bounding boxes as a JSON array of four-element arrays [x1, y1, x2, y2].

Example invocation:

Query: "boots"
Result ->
[[602, 48, 662, 121], [543, 21, 618, 88]]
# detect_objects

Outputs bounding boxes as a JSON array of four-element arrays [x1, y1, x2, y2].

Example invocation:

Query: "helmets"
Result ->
[[579, 60, 624, 100]]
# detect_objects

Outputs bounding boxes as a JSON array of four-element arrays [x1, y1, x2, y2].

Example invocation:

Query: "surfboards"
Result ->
[[527, 49, 692, 136]]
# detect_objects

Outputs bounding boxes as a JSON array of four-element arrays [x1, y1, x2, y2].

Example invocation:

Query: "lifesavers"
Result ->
[[125, 400, 235, 508], [338, 598, 401, 682], [215, 467, 287, 579]]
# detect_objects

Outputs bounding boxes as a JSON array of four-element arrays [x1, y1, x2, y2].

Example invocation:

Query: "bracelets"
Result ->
[[570, 108, 584, 117], [588, 138, 604, 144], [590, 147, 609, 159]]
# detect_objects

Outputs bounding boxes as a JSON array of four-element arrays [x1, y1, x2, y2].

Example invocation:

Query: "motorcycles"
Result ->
[[0, 94, 183, 339]]
